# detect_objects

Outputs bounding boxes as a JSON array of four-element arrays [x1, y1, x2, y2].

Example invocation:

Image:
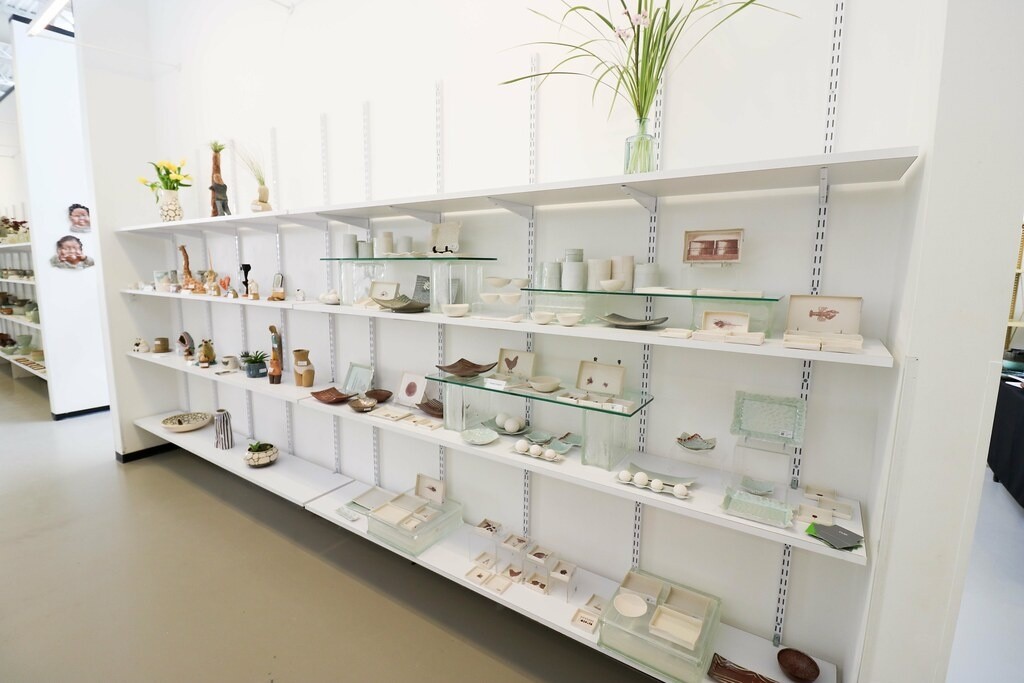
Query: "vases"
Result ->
[[157, 188, 182, 222], [625, 119, 661, 171]]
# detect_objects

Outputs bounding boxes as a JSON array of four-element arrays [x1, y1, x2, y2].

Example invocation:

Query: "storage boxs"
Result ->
[[600, 566, 720, 683], [364, 498, 462, 555]]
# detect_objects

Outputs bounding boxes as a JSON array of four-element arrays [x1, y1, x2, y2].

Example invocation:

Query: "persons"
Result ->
[[49, 235, 95, 270], [68, 203, 92, 233], [208, 173, 232, 217]]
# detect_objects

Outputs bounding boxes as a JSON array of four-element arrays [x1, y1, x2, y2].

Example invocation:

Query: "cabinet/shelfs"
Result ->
[[0, 243, 48, 382], [112, 146, 919, 683]]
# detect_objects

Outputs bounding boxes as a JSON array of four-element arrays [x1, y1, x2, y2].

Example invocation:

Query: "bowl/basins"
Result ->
[[1, 268, 33, 279], [0, 333, 44, 361], [461, 428, 501, 444], [365, 389, 393, 402], [528, 376, 564, 392], [600, 279, 627, 290], [348, 397, 378, 413], [0, 292, 40, 323], [777, 646, 820, 681], [443, 277, 623, 327]]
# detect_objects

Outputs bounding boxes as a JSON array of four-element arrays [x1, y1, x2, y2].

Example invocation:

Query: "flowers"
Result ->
[[141, 157, 192, 202], [499, 1, 800, 163]]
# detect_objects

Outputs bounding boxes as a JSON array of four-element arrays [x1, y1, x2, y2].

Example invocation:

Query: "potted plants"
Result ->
[[242, 351, 269, 378], [245, 441, 278, 468]]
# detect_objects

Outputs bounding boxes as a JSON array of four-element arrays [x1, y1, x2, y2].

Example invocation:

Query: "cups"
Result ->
[[155, 337, 170, 353]]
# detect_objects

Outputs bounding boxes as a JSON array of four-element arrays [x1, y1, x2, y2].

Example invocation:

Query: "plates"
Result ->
[[613, 594, 648, 617], [541, 249, 658, 292], [599, 313, 669, 326], [311, 362, 444, 417], [160, 412, 212, 432], [342, 222, 458, 258], [482, 390, 854, 527], [436, 348, 625, 396], [367, 275, 460, 312]]
